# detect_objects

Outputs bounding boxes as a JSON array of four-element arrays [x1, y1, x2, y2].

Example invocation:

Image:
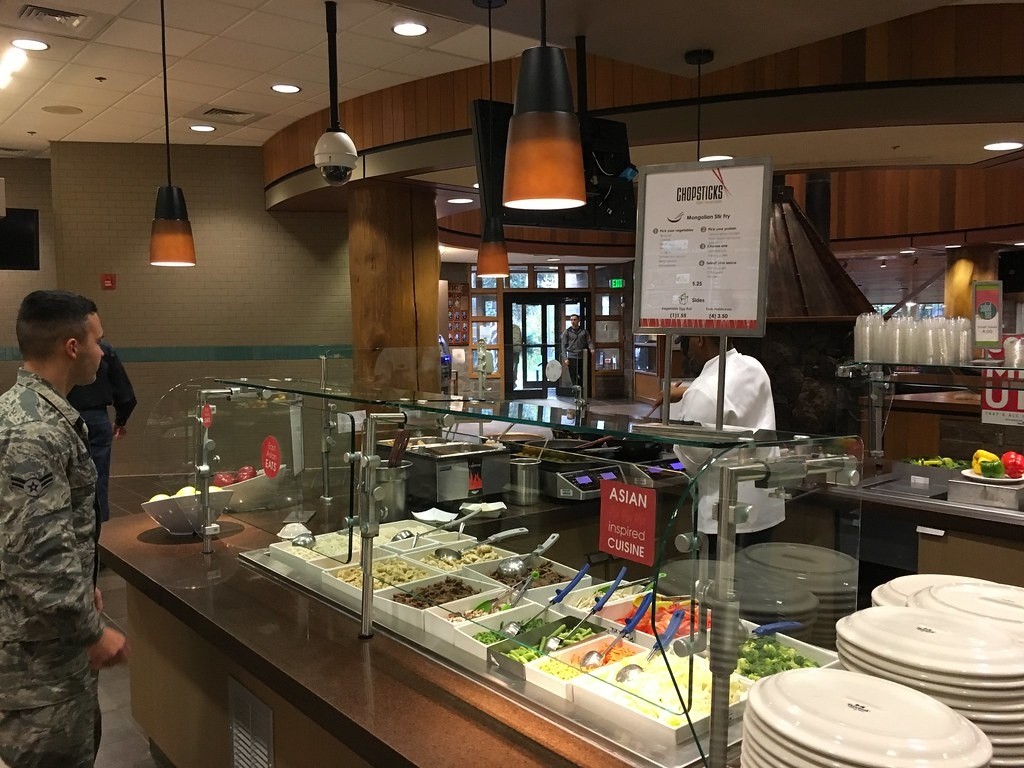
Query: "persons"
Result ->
[[66, 338, 139, 592], [0, 291, 131, 768], [650, 335, 787, 562], [491, 324, 522, 388], [561, 315, 595, 406]]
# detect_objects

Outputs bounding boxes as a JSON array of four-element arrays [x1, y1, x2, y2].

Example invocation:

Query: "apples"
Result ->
[[212, 464, 257, 487]]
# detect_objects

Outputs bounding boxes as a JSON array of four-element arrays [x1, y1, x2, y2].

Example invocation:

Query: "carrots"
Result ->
[[572, 642, 636, 672]]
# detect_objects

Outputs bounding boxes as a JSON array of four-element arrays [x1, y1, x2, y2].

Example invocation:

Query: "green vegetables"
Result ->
[[469, 618, 551, 646]]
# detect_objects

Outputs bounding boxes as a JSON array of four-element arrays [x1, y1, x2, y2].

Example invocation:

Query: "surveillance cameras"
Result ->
[[314, 131, 358, 187]]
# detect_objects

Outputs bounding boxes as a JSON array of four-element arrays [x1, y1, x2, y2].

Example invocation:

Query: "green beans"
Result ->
[[498, 623, 598, 664]]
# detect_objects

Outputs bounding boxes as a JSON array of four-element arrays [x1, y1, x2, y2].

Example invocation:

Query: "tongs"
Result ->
[[592, 572, 666, 600], [472, 574, 533, 613]]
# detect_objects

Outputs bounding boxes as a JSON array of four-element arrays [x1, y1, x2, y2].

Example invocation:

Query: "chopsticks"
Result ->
[[388, 430, 413, 468]]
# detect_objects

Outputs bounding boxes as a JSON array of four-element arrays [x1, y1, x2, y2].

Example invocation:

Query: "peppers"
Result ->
[[611, 603, 713, 641], [972, 449, 1024, 479]]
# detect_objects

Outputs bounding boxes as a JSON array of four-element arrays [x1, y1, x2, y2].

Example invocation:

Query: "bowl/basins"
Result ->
[[853, 311, 973, 366], [1002, 336, 1024, 367], [277, 522, 312, 538], [223, 464, 286, 512], [140, 489, 235, 536]]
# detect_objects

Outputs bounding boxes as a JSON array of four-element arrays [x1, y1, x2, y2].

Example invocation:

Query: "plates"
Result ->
[[660, 542, 858, 647], [960, 468, 1024, 485], [833, 572, 1024, 768], [738, 667, 994, 768]]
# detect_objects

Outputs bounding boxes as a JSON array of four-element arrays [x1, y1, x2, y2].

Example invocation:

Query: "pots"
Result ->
[[481, 432, 546, 445], [525, 439, 622, 458], [376, 436, 510, 504]]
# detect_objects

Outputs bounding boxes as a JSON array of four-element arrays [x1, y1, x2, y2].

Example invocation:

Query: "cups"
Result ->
[[374, 460, 414, 523], [510, 458, 542, 506]]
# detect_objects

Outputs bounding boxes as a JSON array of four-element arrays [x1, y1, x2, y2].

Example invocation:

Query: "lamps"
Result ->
[[502, 0, 587, 209], [469, 0, 510, 278], [149, 0, 197, 266]]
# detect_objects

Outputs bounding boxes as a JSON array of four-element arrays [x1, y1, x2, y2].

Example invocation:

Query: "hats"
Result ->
[[674, 336, 684, 344]]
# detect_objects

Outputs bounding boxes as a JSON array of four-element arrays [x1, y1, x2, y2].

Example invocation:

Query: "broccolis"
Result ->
[[735, 634, 821, 681]]
